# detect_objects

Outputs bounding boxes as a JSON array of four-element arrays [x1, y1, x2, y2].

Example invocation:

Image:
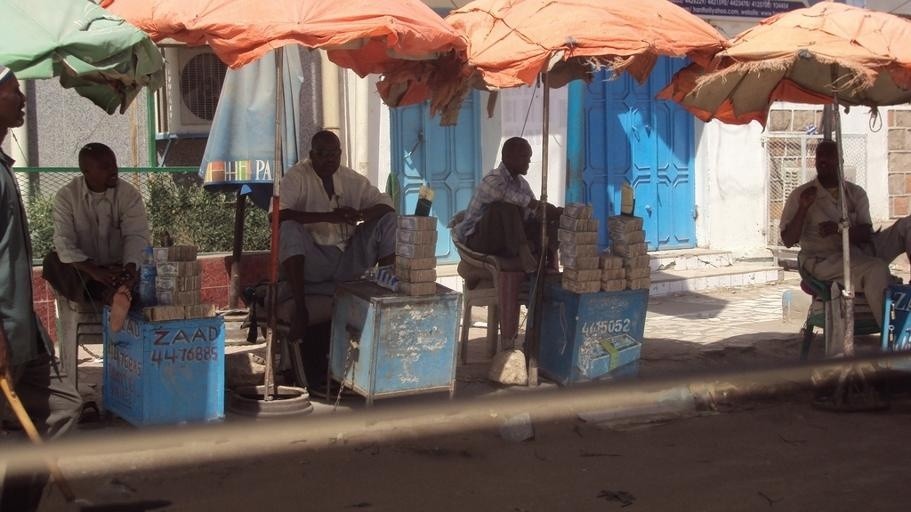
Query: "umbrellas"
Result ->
[[376, 0, 735, 360], [98, 0, 470, 401], [0, 0, 165, 116], [655, 2, 910, 357]]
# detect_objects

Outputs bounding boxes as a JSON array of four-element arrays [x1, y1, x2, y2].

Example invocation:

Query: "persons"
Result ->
[[462, 137, 558, 276], [779, 138, 911, 331], [267, 127, 402, 324], [0, 63, 57, 511], [52, 141, 150, 331]]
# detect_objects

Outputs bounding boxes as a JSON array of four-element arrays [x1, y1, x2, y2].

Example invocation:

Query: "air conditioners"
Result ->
[[150, 43, 226, 165]]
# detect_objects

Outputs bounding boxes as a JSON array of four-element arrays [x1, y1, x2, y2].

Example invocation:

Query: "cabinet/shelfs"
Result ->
[[525, 274, 648, 393], [104, 305, 224, 429], [328, 281, 463, 409]]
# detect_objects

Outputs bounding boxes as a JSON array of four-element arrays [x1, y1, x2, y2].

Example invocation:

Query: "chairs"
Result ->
[[223, 247, 334, 394], [448, 207, 558, 369]]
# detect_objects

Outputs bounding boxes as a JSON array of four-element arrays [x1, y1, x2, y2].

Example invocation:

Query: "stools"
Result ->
[[795, 249, 903, 367], [41, 279, 103, 390]]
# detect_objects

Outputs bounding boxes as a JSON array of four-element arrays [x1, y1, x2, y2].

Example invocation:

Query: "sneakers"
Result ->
[[518, 244, 538, 274], [76, 401, 100, 429], [457, 262, 479, 290]]
